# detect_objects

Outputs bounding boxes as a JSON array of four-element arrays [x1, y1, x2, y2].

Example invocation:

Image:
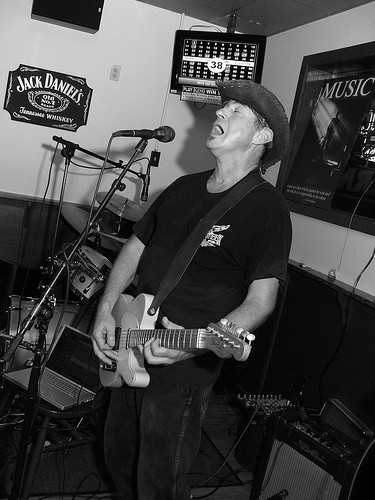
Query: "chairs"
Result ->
[[0, 283, 138, 500]]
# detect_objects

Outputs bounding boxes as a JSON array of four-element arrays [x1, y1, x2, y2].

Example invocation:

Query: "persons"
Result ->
[[90, 79, 292, 500]]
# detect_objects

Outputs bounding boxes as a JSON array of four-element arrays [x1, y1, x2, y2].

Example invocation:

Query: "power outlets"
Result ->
[[109, 65, 121, 81]]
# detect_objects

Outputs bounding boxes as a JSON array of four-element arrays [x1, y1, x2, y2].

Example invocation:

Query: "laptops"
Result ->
[[4, 324, 103, 410]]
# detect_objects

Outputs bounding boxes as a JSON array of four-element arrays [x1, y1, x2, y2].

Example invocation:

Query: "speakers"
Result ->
[[31, 0, 105, 34], [255, 406, 375, 500]]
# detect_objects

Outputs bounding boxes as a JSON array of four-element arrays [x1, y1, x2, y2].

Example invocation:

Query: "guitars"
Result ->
[[98, 292, 257, 387]]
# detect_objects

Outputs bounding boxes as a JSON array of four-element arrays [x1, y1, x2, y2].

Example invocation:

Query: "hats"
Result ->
[[216, 75, 290, 175]]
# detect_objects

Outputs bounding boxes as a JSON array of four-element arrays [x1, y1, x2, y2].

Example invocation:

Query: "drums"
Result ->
[[68, 247, 115, 308]]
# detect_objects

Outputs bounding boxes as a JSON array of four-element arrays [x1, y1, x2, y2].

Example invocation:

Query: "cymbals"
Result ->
[[97, 191, 147, 222], [60, 203, 122, 252]]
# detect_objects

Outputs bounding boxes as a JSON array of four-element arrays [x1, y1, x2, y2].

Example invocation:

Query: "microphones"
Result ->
[[112, 125, 176, 143], [266, 489, 288, 500], [141, 164, 151, 201]]
